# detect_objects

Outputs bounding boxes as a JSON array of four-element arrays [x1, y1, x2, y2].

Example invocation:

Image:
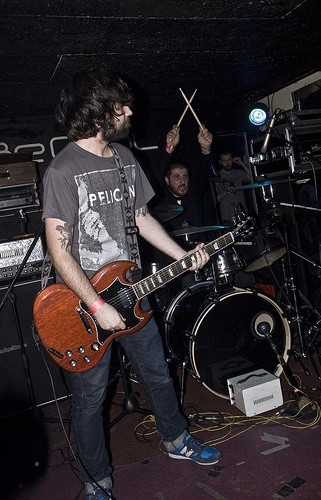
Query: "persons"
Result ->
[[42, 67, 224, 500], [159, 124, 253, 247]]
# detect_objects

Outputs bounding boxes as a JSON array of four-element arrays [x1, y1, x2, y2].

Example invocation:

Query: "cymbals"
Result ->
[[147, 202, 187, 223], [169, 226, 228, 236]]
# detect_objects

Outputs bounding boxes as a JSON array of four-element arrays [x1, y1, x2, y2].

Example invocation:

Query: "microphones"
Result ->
[[251, 312, 275, 340], [261, 114, 276, 153]]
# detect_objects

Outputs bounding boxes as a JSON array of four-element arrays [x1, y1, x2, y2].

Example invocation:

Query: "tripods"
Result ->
[[0, 222, 74, 425], [273, 230, 321, 378], [105, 340, 153, 428]]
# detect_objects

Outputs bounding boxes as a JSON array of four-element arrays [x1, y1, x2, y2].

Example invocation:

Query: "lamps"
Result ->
[[249, 102, 269, 126]]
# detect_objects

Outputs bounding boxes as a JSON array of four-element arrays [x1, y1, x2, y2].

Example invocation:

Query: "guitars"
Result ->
[[33, 212, 258, 371]]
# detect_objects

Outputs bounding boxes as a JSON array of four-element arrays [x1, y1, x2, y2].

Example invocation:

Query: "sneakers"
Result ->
[[86, 487, 112, 500], [168, 434, 222, 466]]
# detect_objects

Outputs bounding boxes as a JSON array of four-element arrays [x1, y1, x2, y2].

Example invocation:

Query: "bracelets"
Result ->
[[88, 297, 105, 314], [167, 143, 176, 151]]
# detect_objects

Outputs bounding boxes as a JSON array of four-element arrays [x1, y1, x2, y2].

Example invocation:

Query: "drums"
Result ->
[[191, 248, 247, 280], [236, 218, 287, 271], [164, 280, 292, 399]]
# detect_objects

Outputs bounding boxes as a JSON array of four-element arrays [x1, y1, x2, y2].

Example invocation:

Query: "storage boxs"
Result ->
[[227, 369, 284, 417]]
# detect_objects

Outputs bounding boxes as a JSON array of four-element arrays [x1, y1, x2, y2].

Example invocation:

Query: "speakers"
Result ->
[[0, 273, 73, 414]]
